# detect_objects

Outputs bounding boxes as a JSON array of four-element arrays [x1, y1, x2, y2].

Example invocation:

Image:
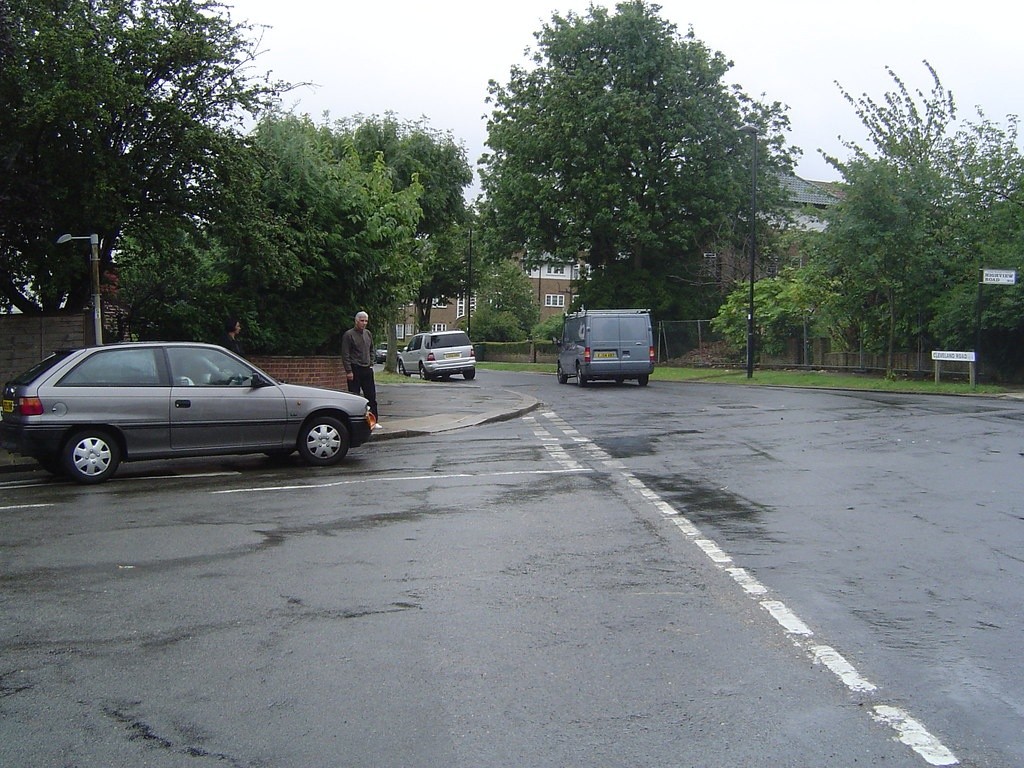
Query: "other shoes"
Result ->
[[375, 424, 382, 429]]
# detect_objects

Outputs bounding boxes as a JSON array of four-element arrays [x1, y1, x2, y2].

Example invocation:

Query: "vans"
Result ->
[[551, 306, 656, 388]]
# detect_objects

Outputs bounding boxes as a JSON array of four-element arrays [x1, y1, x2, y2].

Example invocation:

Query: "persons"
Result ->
[[341, 311, 382, 429], [218, 320, 256, 363]]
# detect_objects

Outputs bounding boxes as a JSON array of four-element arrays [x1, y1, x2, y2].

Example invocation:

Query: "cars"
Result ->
[[0, 339, 383, 485], [376, 342, 403, 364]]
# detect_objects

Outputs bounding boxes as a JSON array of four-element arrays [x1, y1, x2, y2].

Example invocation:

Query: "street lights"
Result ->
[[737, 124, 759, 378], [56, 233, 103, 347]]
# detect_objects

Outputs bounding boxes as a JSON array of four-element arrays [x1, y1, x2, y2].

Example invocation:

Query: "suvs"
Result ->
[[397, 330, 476, 381]]
